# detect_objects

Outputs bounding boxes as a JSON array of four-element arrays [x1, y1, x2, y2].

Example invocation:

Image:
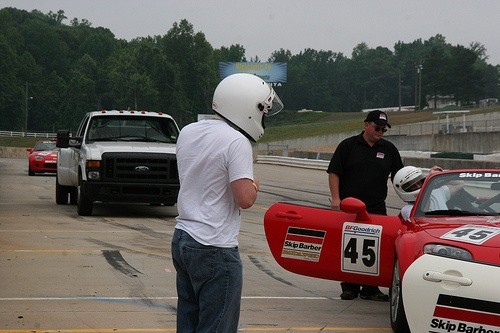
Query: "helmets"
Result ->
[[211, 72, 276, 142], [393, 167, 426, 203]]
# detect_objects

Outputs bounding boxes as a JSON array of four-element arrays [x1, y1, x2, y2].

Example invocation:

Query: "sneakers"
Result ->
[[340, 290, 359, 300], [360, 290, 389, 302]]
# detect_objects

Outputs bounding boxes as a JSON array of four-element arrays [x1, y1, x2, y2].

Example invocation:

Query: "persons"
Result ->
[[170, 72, 284, 333], [326, 109, 405, 302], [392, 165, 464, 221]]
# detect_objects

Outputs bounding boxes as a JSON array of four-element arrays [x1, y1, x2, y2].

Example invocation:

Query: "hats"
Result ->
[[365, 110, 392, 128]]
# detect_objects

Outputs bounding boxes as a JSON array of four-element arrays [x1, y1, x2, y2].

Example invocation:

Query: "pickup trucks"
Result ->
[[55, 108, 180, 215]]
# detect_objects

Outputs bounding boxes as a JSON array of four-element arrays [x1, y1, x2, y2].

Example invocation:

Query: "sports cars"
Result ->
[[263, 167, 499, 333], [26, 139, 58, 176]]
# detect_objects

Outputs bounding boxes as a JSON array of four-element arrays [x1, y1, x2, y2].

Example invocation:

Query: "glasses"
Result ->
[[369, 123, 388, 132]]
